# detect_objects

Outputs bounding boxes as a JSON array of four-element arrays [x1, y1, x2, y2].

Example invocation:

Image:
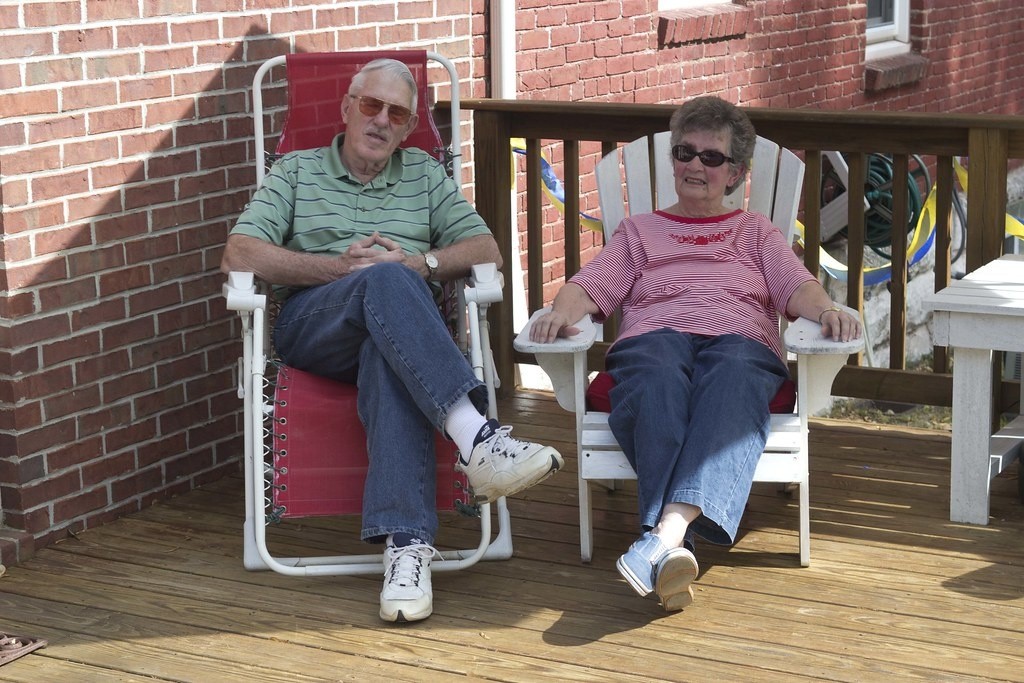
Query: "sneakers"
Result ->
[[456, 418, 564, 504], [379, 532, 444, 622]]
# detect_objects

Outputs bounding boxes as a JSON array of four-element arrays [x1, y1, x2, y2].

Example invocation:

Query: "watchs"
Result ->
[[422, 253, 439, 283], [819, 307, 843, 325]]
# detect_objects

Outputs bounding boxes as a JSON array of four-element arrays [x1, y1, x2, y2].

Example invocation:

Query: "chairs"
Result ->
[[516, 129, 864, 568], [221, 50, 515, 577]]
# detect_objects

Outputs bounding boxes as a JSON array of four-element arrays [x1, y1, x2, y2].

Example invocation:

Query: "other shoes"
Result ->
[[617, 533, 664, 596], [652, 547, 699, 613]]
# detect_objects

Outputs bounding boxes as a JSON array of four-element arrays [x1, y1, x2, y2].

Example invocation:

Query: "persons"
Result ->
[[529, 95, 863, 611], [220, 59, 565, 622]]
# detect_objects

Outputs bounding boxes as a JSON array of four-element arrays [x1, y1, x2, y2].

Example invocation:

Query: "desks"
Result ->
[[921, 252, 1024, 526]]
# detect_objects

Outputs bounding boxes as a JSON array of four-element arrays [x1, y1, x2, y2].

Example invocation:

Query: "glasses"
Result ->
[[672, 145, 734, 168], [350, 93, 414, 125]]
